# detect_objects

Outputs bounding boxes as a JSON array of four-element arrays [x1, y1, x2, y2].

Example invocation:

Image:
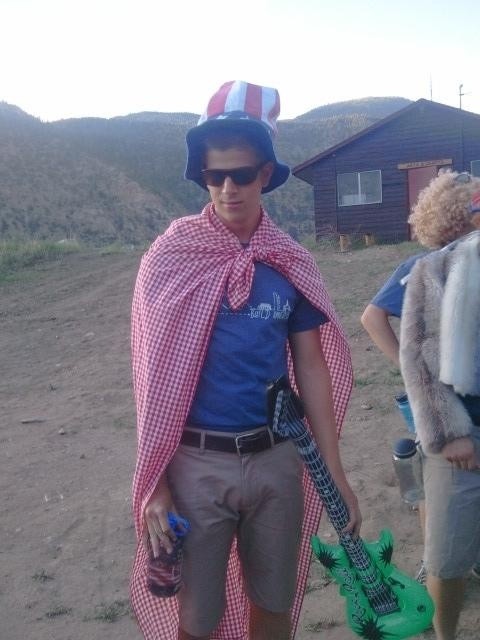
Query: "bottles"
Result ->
[[147, 515, 185, 599], [394, 389, 419, 434], [392, 439, 429, 505]]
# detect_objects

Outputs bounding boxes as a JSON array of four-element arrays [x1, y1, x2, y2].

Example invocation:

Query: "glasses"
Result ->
[[200, 162, 267, 187]]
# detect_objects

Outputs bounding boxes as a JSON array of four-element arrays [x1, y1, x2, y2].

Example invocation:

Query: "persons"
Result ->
[[128, 78, 360, 640], [361, 163, 479, 588], [399, 230, 478, 640]]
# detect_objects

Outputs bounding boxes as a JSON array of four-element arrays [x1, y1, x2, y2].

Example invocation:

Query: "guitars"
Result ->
[[266, 374, 435, 639]]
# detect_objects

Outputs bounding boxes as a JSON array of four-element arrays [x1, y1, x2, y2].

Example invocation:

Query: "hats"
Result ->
[[183, 81, 291, 195]]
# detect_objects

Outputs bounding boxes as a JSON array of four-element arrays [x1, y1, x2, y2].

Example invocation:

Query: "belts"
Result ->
[[179, 429, 288, 454]]
